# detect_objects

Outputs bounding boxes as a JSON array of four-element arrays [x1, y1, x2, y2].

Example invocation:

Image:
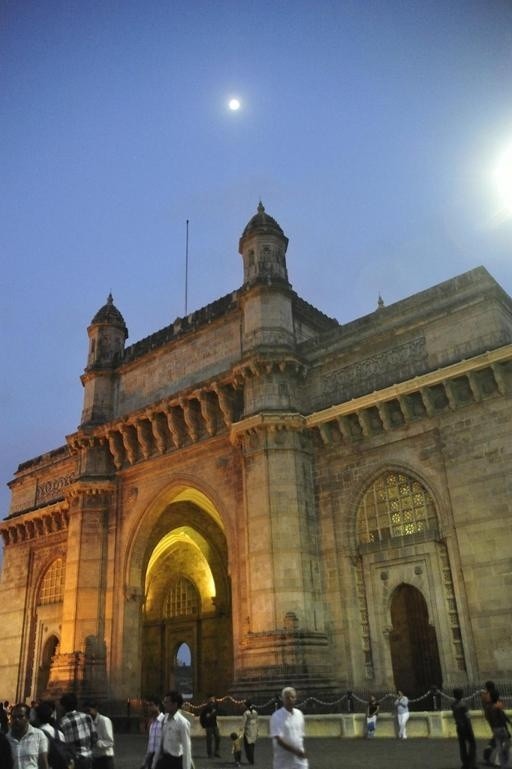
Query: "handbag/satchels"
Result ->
[[156, 751, 182, 768]]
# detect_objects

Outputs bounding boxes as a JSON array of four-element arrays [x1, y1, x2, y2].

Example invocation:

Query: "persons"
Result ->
[[240, 702, 258, 764], [0, 693, 115, 769], [367, 695, 379, 738], [141, 697, 166, 769], [231, 728, 244, 767], [452, 680, 512, 769], [270, 687, 311, 769], [394, 690, 409, 740], [200, 701, 221, 759], [159, 691, 195, 769]]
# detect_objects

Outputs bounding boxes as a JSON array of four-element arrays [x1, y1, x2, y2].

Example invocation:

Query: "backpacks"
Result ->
[[42, 728, 71, 768]]
